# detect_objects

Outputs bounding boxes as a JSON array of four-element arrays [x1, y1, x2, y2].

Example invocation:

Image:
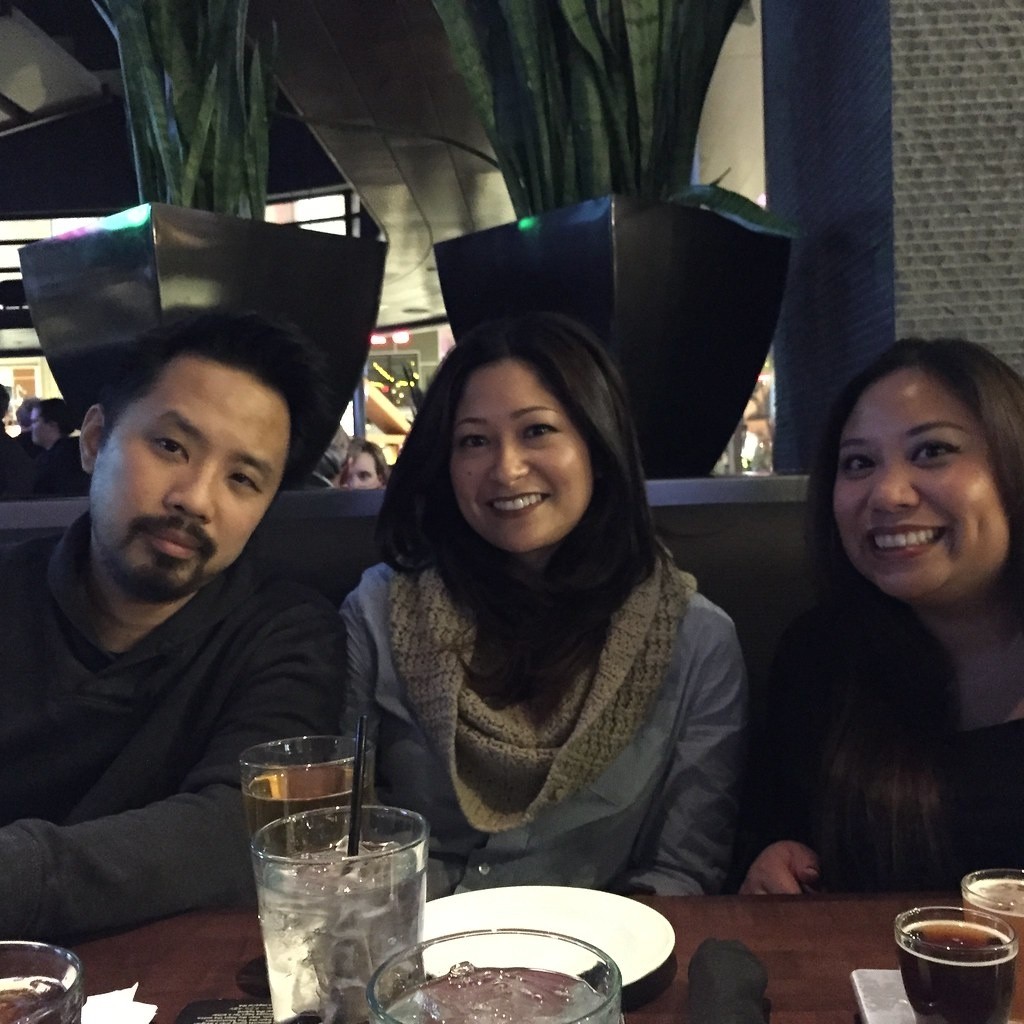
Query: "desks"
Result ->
[[0, 892, 963, 1024]]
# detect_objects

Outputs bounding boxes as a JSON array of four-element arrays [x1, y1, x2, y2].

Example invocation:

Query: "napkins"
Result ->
[[81, 981, 158, 1024]]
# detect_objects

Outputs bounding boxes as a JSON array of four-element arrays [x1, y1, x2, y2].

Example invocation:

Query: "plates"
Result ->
[[425, 884, 675, 987]]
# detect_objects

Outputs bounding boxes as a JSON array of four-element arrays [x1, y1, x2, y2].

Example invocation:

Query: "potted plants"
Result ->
[[15, 0, 401, 483], [412, 0, 812, 479]]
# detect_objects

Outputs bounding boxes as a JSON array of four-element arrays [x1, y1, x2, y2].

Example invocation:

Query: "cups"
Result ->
[[961, 869, 1024, 1024], [894, 905, 1019, 1024], [247, 805, 430, 1024], [0, 940, 85, 1024], [365, 928, 625, 1024], [238, 736, 376, 840]]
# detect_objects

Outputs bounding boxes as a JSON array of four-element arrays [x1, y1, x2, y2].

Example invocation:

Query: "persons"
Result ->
[[0, 376, 776, 497], [730, 333, 1024, 899], [279, 313, 749, 901], [1, 310, 343, 948]]
